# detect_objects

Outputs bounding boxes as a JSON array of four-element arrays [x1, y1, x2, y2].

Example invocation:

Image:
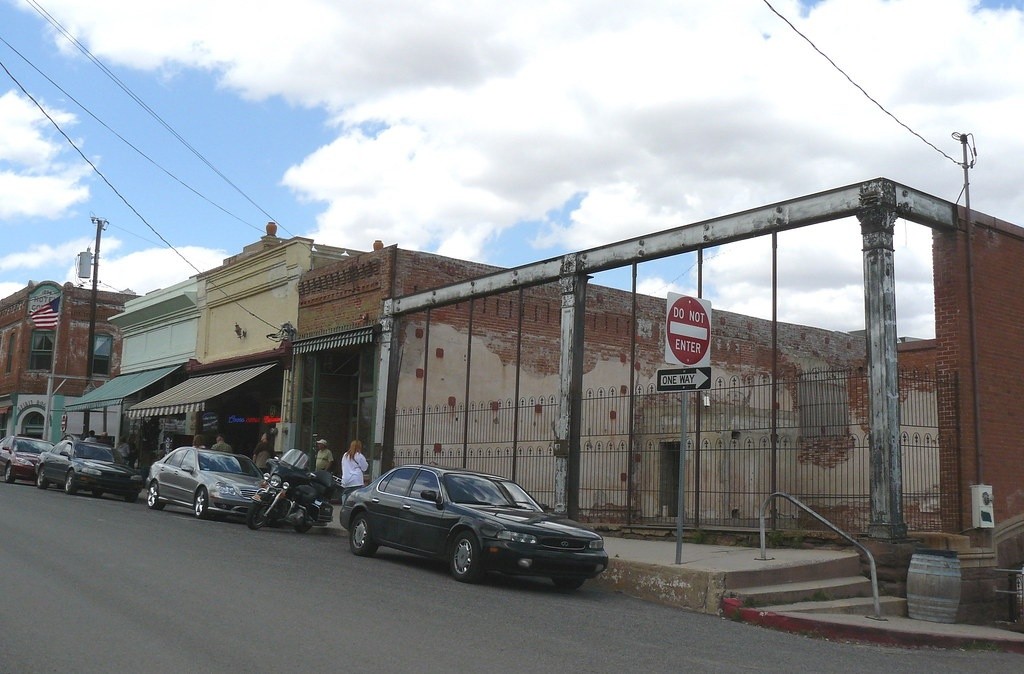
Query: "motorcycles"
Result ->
[[246, 447, 348, 535]]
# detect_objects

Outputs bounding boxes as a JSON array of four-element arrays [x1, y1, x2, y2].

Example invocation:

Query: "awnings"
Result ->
[[124, 361, 281, 419], [64, 365, 184, 413]]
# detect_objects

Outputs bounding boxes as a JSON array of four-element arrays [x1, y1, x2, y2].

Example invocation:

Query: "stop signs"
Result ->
[[664, 291, 713, 367], [60, 415, 67, 432]]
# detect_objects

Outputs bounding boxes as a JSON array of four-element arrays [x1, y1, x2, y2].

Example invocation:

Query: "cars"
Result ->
[[60, 433, 114, 444], [33, 439, 145, 503], [337, 462, 609, 590], [144, 446, 268, 519], [0, 435, 56, 485]]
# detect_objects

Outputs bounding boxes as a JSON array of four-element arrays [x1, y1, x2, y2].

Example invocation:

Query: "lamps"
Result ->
[[234, 322, 245, 339]]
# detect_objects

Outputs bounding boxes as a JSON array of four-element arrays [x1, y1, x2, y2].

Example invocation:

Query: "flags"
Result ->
[[30, 294, 61, 331]]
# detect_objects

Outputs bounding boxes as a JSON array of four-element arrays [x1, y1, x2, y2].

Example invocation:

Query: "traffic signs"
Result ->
[[656, 367, 713, 392]]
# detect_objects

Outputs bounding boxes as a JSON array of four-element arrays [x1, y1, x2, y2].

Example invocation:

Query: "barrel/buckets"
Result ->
[[906, 548, 962, 623]]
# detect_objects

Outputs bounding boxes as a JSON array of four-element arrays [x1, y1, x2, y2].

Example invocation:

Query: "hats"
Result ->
[[317, 439, 327, 445]]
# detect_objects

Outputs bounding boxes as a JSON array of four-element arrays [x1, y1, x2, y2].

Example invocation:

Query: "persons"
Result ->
[[82, 430, 370, 507]]
